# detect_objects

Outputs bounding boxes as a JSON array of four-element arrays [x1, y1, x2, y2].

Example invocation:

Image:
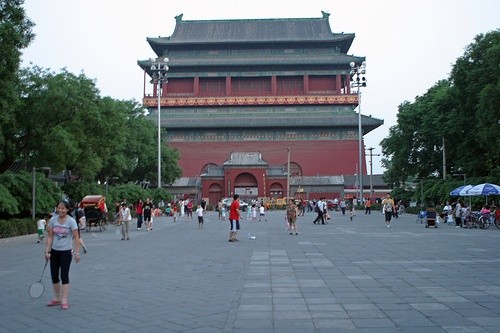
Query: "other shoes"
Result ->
[[61, 304, 69, 309], [47, 300, 62, 306]]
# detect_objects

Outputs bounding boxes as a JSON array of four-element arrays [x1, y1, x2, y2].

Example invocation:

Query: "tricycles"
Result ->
[[78, 194, 108, 232]]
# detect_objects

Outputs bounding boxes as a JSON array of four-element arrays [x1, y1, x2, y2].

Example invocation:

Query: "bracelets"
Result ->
[[76, 253, 80, 255]]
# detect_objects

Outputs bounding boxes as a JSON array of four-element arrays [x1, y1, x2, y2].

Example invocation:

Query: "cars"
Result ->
[[265, 196, 337, 211], [221, 197, 249, 212]]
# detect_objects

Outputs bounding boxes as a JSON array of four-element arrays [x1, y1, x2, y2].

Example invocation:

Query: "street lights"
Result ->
[[348, 60, 367, 205], [148, 56, 170, 188]]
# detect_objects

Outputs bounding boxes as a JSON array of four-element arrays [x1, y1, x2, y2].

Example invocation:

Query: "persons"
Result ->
[[142, 197, 153, 231], [36, 215, 46, 243], [298, 197, 371, 225], [114, 202, 122, 225], [201, 200, 207, 212], [216, 199, 225, 220], [381, 193, 500, 228], [153, 205, 177, 222], [178, 197, 193, 218], [68, 200, 87, 253], [135, 198, 144, 230], [286, 199, 298, 235], [247, 199, 266, 222], [195, 205, 204, 228], [114, 202, 131, 240], [229, 194, 242, 242], [45, 201, 80, 309]]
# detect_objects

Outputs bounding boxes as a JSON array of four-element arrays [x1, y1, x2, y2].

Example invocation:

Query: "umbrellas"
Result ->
[[450, 185, 476, 210], [466, 183, 500, 205]]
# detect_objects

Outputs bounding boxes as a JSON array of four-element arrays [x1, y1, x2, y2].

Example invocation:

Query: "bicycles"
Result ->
[[478, 212, 500, 230]]
